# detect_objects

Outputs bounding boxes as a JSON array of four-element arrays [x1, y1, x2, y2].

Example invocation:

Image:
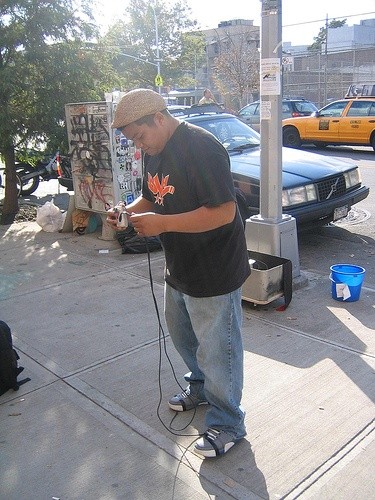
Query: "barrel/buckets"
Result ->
[[329, 264, 366, 302]]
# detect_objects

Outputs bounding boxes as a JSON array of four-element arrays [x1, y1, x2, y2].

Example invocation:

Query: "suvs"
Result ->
[[236, 99, 318, 133]]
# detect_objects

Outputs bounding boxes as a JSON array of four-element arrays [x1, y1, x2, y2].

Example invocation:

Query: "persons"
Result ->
[[198, 88, 217, 105], [105, 88, 252, 457]]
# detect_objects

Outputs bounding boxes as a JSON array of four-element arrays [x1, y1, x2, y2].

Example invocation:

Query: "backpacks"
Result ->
[[0, 319, 31, 397]]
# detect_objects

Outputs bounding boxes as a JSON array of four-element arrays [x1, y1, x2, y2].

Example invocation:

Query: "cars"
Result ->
[[282, 98, 375, 152], [57, 106, 369, 233]]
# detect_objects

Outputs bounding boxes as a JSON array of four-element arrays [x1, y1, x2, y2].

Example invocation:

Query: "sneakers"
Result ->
[[192, 428, 236, 457], [168, 385, 211, 412]]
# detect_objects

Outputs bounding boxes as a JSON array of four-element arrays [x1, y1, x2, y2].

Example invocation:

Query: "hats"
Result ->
[[111, 88, 167, 129]]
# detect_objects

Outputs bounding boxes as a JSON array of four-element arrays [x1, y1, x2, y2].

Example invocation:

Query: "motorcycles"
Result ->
[[15, 148, 60, 196]]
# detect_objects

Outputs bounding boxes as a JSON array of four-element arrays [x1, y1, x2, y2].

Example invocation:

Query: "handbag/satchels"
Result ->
[[36, 198, 64, 232], [114, 227, 162, 255]]
